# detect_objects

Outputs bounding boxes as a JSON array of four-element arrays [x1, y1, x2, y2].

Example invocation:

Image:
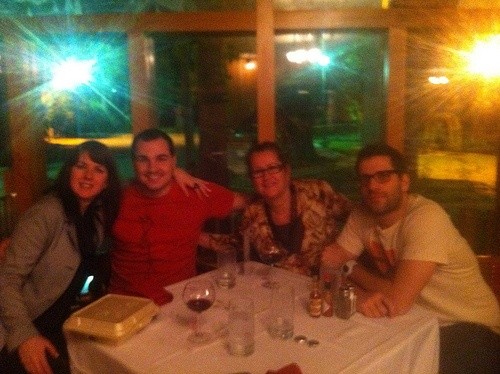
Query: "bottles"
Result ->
[[337, 284, 356, 319], [321, 273, 334, 317], [309, 275, 321, 317]]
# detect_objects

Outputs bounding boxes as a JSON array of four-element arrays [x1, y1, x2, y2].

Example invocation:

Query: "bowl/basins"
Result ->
[[63, 293, 160, 345]]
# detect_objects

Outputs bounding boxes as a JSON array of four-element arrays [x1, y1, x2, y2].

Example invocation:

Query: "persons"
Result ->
[[197, 141, 360, 276], [108, 129, 260, 297], [0, 140, 212, 374], [319, 144, 500, 374]]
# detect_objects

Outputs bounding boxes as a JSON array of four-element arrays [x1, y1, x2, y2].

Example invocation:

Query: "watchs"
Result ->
[[341, 260, 358, 277]]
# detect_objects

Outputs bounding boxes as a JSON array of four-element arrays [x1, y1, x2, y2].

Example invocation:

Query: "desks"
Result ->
[[64, 261, 440, 374]]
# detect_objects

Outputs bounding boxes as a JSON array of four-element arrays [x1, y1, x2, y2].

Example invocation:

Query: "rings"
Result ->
[[193, 183, 199, 189]]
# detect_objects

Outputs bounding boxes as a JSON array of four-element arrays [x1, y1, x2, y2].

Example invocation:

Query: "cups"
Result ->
[[269, 284, 296, 339], [229, 298, 255, 356], [216, 244, 237, 288]]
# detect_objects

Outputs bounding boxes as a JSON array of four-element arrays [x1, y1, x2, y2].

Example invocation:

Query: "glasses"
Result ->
[[353, 169, 401, 187], [249, 163, 287, 179]]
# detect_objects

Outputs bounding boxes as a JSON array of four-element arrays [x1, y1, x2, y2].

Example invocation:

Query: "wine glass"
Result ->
[[257, 240, 285, 288], [183, 280, 215, 344]]
